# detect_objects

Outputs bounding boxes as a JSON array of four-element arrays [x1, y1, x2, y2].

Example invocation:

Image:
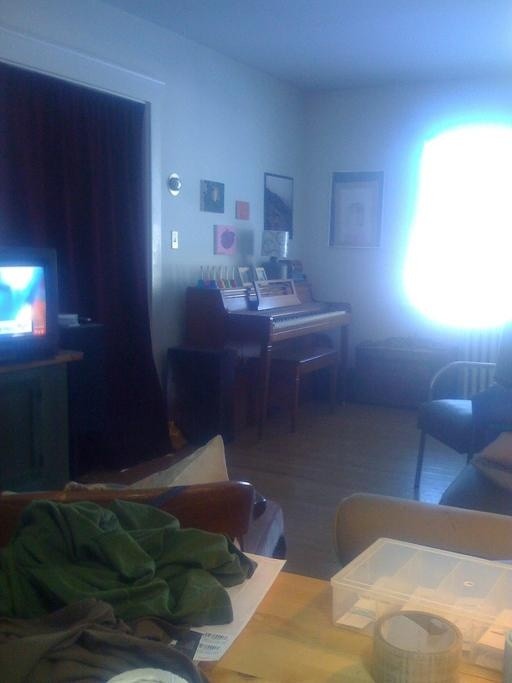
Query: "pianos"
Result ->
[[166, 279, 352, 443]]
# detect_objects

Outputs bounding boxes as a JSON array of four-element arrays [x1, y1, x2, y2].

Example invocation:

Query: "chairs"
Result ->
[[334, 426, 512, 562], [0, 477, 291, 569], [411, 334, 512, 488]]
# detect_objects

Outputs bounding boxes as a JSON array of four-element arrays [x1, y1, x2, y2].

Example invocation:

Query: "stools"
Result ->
[[274, 346, 342, 432]]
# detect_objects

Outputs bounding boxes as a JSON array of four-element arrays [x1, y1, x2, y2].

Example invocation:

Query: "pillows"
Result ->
[[470, 432, 512, 499], [68, 430, 229, 492]]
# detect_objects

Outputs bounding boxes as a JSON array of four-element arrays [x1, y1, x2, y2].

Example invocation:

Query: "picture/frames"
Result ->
[[328, 169, 385, 249], [262, 172, 295, 240]]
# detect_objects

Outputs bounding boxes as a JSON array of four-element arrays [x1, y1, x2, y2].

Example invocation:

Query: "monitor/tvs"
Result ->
[[0, 245, 63, 366]]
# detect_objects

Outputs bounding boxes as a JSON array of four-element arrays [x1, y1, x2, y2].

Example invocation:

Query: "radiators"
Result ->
[[461, 330, 504, 400]]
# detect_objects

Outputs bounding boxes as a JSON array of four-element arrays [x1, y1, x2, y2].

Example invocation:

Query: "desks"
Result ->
[[0, 348, 88, 490], [165, 340, 265, 446], [186, 567, 502, 683]]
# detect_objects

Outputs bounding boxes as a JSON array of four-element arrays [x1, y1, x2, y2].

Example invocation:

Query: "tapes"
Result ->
[[372, 610, 463, 683]]
